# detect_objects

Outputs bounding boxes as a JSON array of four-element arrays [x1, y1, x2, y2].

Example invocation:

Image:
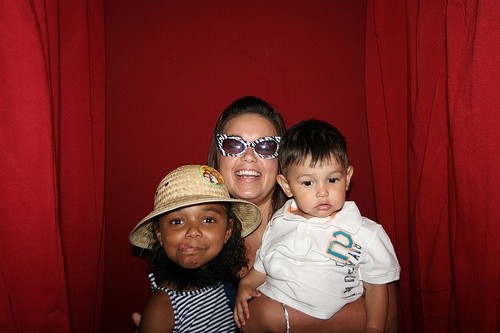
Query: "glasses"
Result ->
[[217, 133, 282, 160]]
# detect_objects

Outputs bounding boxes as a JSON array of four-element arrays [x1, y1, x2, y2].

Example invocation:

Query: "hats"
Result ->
[[128, 165, 263, 250]]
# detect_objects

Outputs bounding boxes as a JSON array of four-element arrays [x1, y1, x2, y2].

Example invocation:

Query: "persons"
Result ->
[[134, 164, 261, 333], [131, 96, 400, 333], [233, 118, 401, 333]]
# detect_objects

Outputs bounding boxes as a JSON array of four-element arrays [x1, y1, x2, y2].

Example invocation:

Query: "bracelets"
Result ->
[[280, 301, 291, 333]]
